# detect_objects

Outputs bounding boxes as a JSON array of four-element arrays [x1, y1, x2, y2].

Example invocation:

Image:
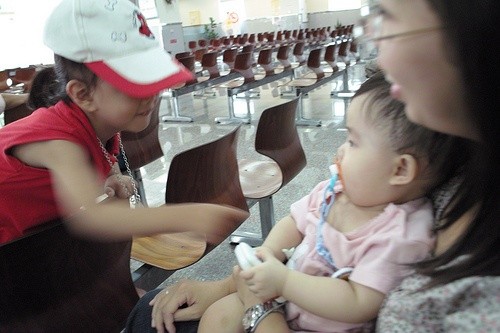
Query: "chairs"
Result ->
[[0, 24, 407, 270]]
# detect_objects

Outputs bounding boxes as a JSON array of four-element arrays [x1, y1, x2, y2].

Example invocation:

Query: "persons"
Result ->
[[128, 0, 500, 333], [0, 0, 250, 333], [197, 67, 470, 333]]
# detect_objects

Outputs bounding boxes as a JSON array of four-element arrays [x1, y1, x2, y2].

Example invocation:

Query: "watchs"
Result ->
[[242, 301, 285, 333]]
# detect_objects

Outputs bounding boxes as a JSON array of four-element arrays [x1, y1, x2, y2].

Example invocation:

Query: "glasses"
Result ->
[[351, 0, 455, 60]]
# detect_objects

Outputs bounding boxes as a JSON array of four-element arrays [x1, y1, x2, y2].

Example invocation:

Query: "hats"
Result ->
[[40, 1, 196, 98]]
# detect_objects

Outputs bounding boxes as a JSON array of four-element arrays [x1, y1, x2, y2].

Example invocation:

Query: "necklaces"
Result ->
[[96, 130, 141, 210]]
